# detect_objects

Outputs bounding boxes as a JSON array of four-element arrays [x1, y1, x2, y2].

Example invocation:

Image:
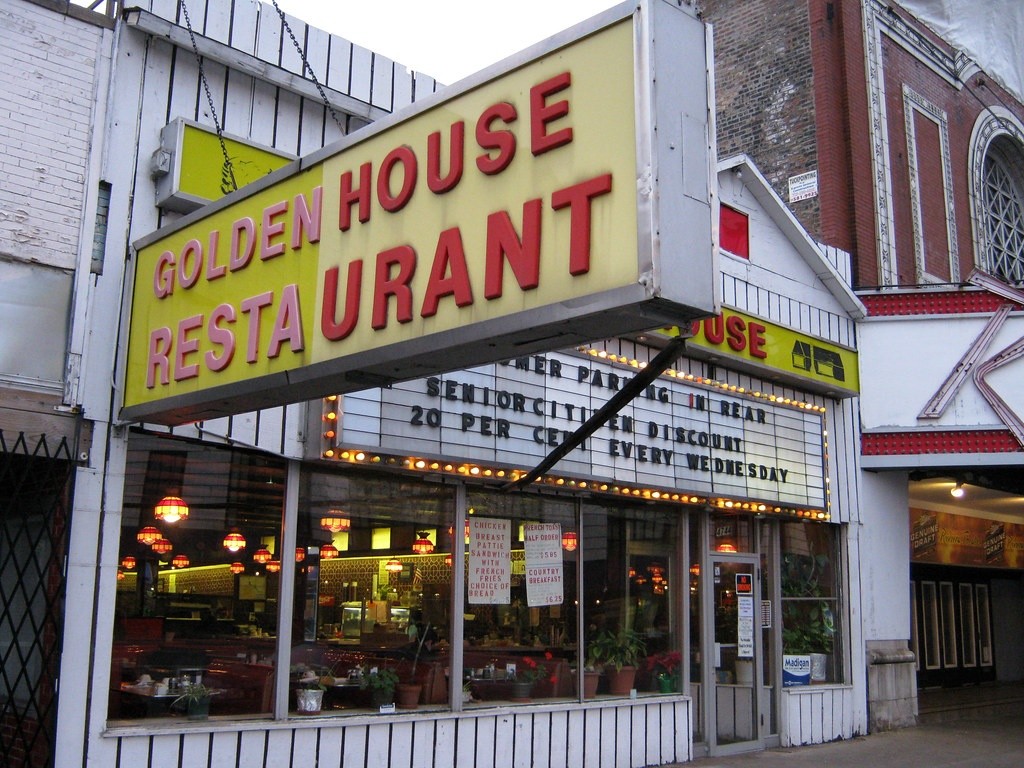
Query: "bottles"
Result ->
[[483, 664, 494, 677]]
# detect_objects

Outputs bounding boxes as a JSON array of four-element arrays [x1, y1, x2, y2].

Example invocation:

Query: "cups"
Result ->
[[155, 673, 191, 694]]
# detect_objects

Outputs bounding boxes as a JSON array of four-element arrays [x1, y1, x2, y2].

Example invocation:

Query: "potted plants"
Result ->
[[357, 665, 397, 711], [463, 679, 473, 704], [396, 590, 452, 709], [784, 552, 838, 684], [294, 663, 330, 717], [170, 681, 216, 721], [568, 656, 603, 699], [592, 622, 648, 694]]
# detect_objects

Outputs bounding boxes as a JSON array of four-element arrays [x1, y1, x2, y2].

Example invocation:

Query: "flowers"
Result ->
[[644, 648, 682, 673], [503, 650, 561, 685]]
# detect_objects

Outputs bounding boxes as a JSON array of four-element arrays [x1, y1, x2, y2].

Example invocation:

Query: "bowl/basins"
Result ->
[[139, 674, 151, 680]]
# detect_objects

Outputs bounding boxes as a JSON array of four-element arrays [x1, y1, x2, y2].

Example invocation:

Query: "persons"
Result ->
[[148, 624, 186, 665], [394, 628, 438, 660]]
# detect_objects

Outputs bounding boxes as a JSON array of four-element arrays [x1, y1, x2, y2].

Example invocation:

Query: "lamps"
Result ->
[[295, 546, 306, 563], [221, 527, 247, 553], [411, 530, 437, 555], [252, 543, 273, 564], [121, 554, 136, 570], [384, 556, 404, 574], [136, 525, 163, 546], [148, 537, 173, 554], [171, 553, 191, 569], [317, 543, 339, 560], [229, 559, 246, 575], [152, 494, 190, 525], [562, 527, 578, 552], [265, 559, 280, 574], [319, 503, 351, 533]]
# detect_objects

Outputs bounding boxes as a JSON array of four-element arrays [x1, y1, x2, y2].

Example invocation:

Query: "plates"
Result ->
[[137, 679, 155, 684]]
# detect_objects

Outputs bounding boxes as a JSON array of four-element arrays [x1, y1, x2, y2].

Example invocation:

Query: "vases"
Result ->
[[509, 682, 532, 698], [657, 672, 676, 694]]
